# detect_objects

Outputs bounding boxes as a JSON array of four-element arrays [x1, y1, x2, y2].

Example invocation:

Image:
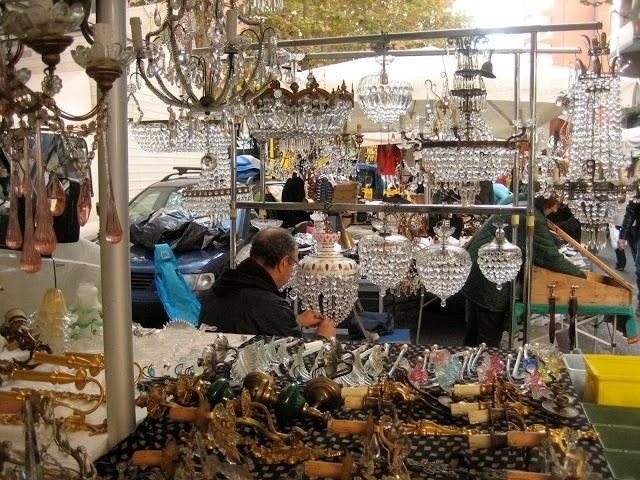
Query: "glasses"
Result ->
[[291, 257, 304, 271]]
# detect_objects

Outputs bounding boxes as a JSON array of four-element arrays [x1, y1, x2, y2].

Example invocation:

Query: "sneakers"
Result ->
[[636, 300, 640, 316], [615, 262, 624, 271]]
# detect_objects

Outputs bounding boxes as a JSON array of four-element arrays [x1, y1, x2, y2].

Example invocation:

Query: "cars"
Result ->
[[0, 200, 102, 332], [90, 168, 287, 323]]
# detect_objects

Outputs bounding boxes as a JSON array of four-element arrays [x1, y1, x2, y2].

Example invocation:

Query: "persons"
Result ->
[[617, 190, 640, 319], [282, 168, 314, 232], [460, 192, 583, 346], [196, 229, 337, 340], [612, 190, 632, 273]]
